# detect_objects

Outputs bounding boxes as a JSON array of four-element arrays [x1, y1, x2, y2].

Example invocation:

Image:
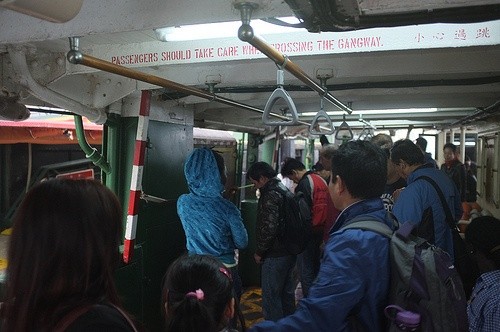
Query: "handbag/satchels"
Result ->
[[453, 228, 471, 281]]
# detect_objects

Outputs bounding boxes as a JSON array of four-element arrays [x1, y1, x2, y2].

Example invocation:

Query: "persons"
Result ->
[[5, 179, 142, 332], [247, 134, 500, 332], [177, 148, 250, 321], [160, 254, 244, 332]]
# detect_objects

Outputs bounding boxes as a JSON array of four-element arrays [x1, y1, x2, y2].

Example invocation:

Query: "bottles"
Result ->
[[384, 305, 421, 332]]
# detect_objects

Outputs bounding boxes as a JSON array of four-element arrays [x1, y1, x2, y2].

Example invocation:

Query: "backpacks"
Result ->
[[339, 210, 468, 332], [259, 185, 312, 256]]
